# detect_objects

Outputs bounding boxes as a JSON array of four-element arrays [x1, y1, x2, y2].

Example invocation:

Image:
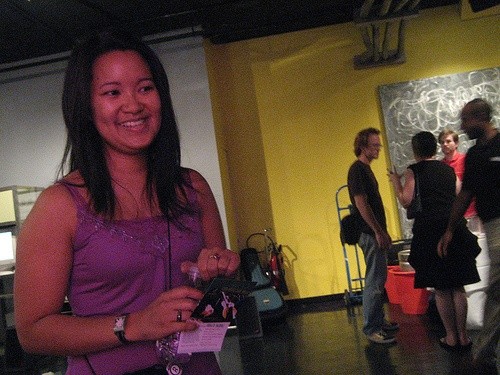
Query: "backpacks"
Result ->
[[239, 247, 288, 322]]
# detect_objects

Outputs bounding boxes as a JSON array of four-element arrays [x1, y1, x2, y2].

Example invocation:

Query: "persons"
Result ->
[[438, 130, 481, 236], [347, 128, 400, 343], [437, 99, 500, 375], [386, 132, 482, 348], [13, 29, 241, 375]]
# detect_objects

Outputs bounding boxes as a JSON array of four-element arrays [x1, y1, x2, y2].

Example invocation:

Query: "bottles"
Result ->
[[156, 266, 205, 363]]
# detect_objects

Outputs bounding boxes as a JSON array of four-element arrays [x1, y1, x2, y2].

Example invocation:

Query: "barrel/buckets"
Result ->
[[385, 266, 404, 304], [390, 267, 430, 315]]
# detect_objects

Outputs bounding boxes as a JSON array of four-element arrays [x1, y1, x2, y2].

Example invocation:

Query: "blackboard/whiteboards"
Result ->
[[377, 67, 500, 241]]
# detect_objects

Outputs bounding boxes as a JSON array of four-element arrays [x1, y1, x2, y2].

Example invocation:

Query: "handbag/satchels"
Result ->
[[406, 163, 423, 220], [340, 215, 362, 245]]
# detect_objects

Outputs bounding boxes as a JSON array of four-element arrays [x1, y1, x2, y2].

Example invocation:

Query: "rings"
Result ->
[[177, 309, 181, 322], [208, 254, 220, 259], [390, 172, 393, 174]]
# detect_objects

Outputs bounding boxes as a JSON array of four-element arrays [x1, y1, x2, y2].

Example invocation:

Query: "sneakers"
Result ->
[[366, 327, 397, 343], [380, 319, 400, 330]]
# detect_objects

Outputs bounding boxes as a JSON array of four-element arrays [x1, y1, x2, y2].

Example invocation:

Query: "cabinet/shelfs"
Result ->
[[0, 184, 47, 375]]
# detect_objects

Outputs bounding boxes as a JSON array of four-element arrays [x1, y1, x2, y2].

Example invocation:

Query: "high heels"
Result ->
[[458, 336, 472, 351], [439, 335, 458, 351]]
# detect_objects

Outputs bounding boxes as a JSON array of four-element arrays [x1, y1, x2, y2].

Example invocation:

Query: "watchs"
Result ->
[[113, 313, 130, 346]]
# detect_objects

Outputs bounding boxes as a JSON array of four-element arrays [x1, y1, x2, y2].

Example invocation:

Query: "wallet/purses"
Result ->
[[173, 266, 231, 354]]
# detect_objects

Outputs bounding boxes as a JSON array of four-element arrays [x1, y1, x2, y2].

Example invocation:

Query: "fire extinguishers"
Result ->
[[267, 245, 284, 293]]
